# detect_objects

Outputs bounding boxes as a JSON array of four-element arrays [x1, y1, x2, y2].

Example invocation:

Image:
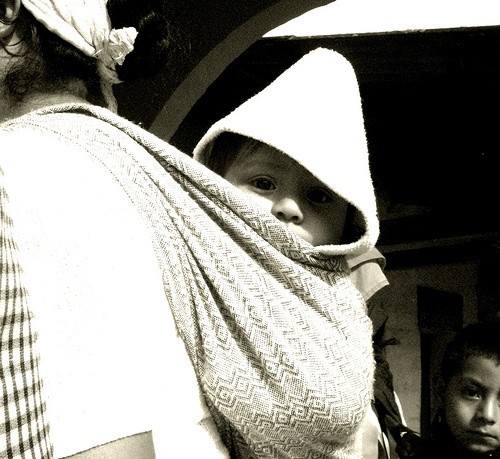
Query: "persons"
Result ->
[[420, 321, 500, 459], [190, 47, 379, 459], [0, 0, 378, 459]]
